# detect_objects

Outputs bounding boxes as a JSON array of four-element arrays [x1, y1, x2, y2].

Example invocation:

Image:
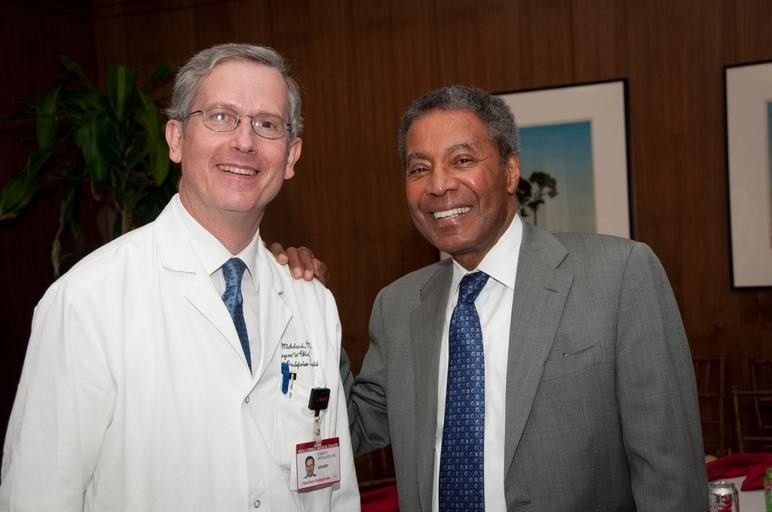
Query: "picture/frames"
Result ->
[[484, 76, 636, 245], [722, 59, 771, 290]]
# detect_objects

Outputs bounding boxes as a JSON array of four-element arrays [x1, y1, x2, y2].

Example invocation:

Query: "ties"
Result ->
[[223, 259, 251, 369], [437, 273, 489, 511]]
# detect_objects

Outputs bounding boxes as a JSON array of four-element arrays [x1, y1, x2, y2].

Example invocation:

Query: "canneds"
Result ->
[[709, 481, 740, 512], [763, 469, 772, 512]]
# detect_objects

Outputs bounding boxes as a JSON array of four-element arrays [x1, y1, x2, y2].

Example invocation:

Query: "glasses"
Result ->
[[180, 106, 294, 139]]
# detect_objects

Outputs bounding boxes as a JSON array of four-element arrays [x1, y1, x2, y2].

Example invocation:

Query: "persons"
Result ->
[[270, 82, 712, 512], [302, 455, 318, 478], [2, 41, 366, 511]]
